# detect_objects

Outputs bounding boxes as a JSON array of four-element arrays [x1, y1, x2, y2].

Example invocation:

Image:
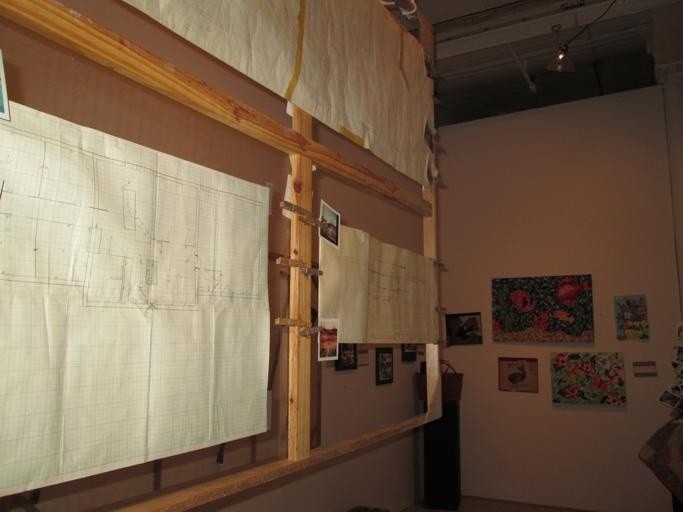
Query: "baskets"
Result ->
[[415, 360, 463, 403]]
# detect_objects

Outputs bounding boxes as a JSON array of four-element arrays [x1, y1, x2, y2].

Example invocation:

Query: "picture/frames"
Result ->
[[399, 343, 415, 362], [334, 344, 358, 371], [373, 344, 393, 386]]
